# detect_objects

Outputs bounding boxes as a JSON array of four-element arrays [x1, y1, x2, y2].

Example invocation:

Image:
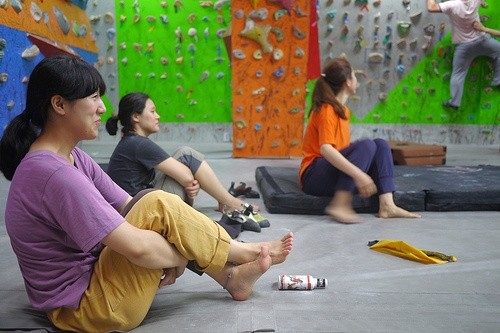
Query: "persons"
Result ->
[[427, 0, 500, 109], [106, 92, 260, 215], [299, 59, 422, 223], [473, 21, 500, 36], [0, 54, 294, 333]]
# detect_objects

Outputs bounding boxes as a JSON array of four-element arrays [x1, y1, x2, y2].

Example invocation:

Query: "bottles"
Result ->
[[278, 274, 327, 290]]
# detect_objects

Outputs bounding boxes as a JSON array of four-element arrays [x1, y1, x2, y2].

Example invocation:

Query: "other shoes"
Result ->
[[228, 181, 260, 199]]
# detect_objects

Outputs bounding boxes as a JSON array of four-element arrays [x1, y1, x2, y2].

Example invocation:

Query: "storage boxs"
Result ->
[[388, 140, 447, 166]]
